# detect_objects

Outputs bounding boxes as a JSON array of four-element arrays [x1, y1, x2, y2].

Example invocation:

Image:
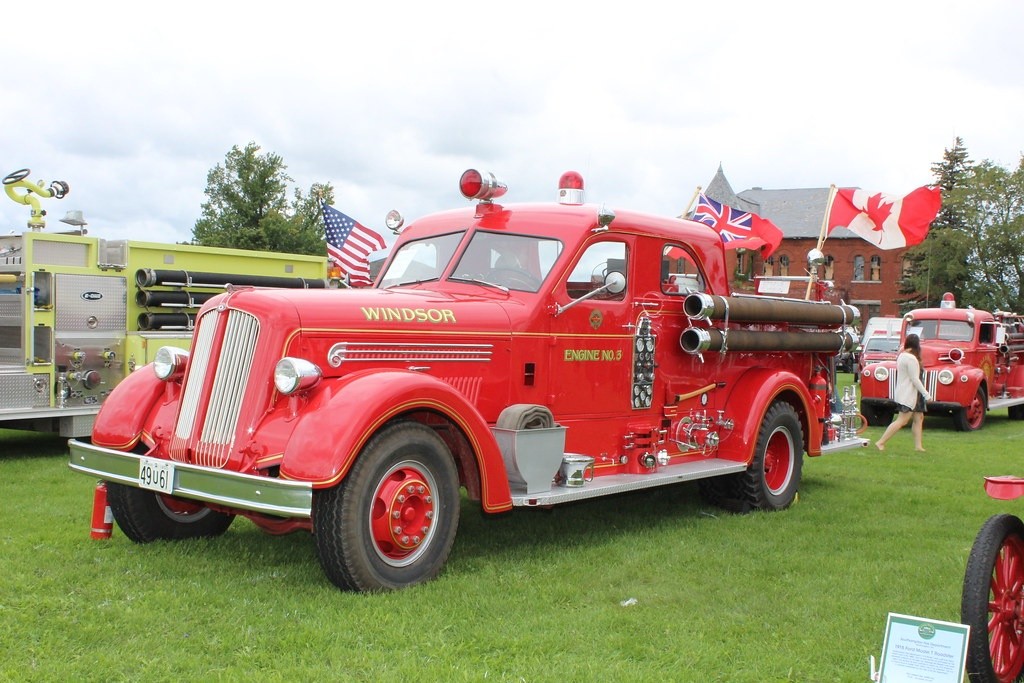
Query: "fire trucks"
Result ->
[[0, 167, 353, 447]]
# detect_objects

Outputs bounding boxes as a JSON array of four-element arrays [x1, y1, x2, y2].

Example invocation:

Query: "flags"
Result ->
[[825, 185, 940, 250], [691, 193, 752, 252], [320, 202, 386, 286]]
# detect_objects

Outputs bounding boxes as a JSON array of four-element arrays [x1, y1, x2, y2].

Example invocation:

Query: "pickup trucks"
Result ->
[[66, 168, 863, 593], [859, 293, 1022, 431]]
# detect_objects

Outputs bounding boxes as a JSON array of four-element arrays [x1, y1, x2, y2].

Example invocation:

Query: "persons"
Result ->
[[875, 334, 932, 452]]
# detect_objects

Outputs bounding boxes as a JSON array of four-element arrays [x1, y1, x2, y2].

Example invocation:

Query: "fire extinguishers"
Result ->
[[91, 479, 115, 540]]
